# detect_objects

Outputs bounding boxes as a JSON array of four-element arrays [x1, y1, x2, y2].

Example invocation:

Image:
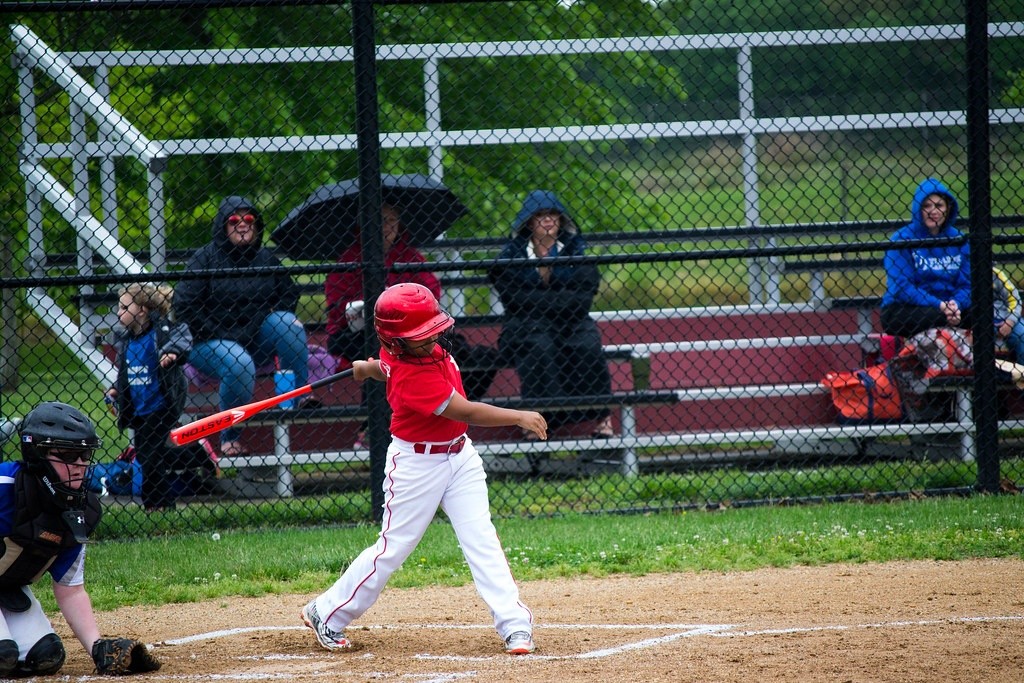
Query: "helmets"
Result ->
[[373, 283, 455, 364], [22, 401, 103, 505]]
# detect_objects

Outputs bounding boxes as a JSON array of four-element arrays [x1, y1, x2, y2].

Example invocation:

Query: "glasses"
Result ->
[[43, 449, 93, 465], [227, 214, 256, 226], [922, 203, 944, 209]]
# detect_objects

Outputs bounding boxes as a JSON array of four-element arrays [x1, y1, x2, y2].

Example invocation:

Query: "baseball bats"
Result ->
[[168, 367, 351, 452]]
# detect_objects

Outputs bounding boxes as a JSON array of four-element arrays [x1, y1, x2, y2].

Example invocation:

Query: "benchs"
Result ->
[[165, 297, 1024, 488]]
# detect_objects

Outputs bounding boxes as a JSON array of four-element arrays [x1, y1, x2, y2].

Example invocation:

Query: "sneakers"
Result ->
[[505, 630, 535, 654], [301, 599, 351, 652]]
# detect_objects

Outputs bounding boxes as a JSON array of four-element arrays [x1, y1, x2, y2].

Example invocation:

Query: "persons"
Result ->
[[881, 178, 1024, 421], [169, 194, 321, 454], [0, 402, 160, 680], [486, 190, 613, 436], [298, 282, 548, 654], [104, 283, 217, 512], [324, 200, 442, 451]]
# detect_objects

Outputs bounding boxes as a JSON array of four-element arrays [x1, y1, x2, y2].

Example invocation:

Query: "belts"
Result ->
[[414, 437, 466, 454]]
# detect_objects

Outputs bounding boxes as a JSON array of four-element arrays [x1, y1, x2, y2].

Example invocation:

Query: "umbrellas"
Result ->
[[270, 174, 471, 262]]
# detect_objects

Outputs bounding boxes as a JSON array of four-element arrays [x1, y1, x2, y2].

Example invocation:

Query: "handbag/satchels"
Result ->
[[821, 362, 908, 424]]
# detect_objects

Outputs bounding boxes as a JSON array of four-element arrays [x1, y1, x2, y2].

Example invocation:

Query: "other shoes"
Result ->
[[299, 396, 322, 410], [220, 442, 249, 458], [592, 431, 614, 439]]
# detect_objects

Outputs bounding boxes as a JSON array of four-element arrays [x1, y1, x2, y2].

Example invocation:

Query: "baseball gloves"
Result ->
[[92, 636, 163, 677]]
